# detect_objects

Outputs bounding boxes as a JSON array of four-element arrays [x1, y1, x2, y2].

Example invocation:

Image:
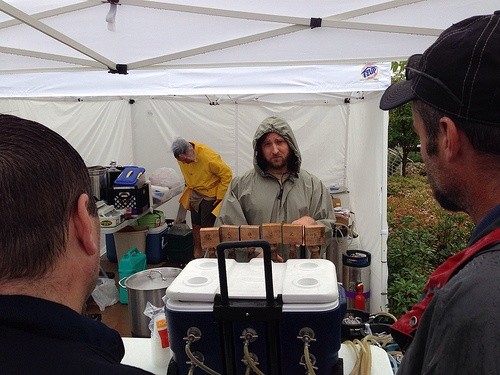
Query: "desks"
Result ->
[[120, 337, 393, 375]]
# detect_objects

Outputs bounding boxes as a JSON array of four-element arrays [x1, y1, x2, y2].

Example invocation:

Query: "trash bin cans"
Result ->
[[343, 250, 371, 314]]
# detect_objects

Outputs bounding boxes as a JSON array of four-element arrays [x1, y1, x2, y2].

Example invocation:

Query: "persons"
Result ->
[[0, 113, 155, 375], [203, 115, 336, 262], [379, 11, 500, 375], [170, 138, 232, 259]]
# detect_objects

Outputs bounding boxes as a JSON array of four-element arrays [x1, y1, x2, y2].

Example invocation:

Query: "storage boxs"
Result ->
[[161, 240, 347, 375], [113, 185, 150, 215], [105, 223, 168, 265], [154, 183, 185, 204]]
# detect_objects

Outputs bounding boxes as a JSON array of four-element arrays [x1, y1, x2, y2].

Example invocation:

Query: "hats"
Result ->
[[379, 10, 500, 125]]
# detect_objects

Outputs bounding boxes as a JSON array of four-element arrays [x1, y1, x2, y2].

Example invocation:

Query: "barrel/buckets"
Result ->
[[88, 168, 107, 201], [118, 266, 182, 338], [118, 247, 147, 305]]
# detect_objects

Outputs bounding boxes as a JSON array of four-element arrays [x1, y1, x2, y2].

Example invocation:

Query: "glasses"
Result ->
[[405, 53, 464, 108]]
